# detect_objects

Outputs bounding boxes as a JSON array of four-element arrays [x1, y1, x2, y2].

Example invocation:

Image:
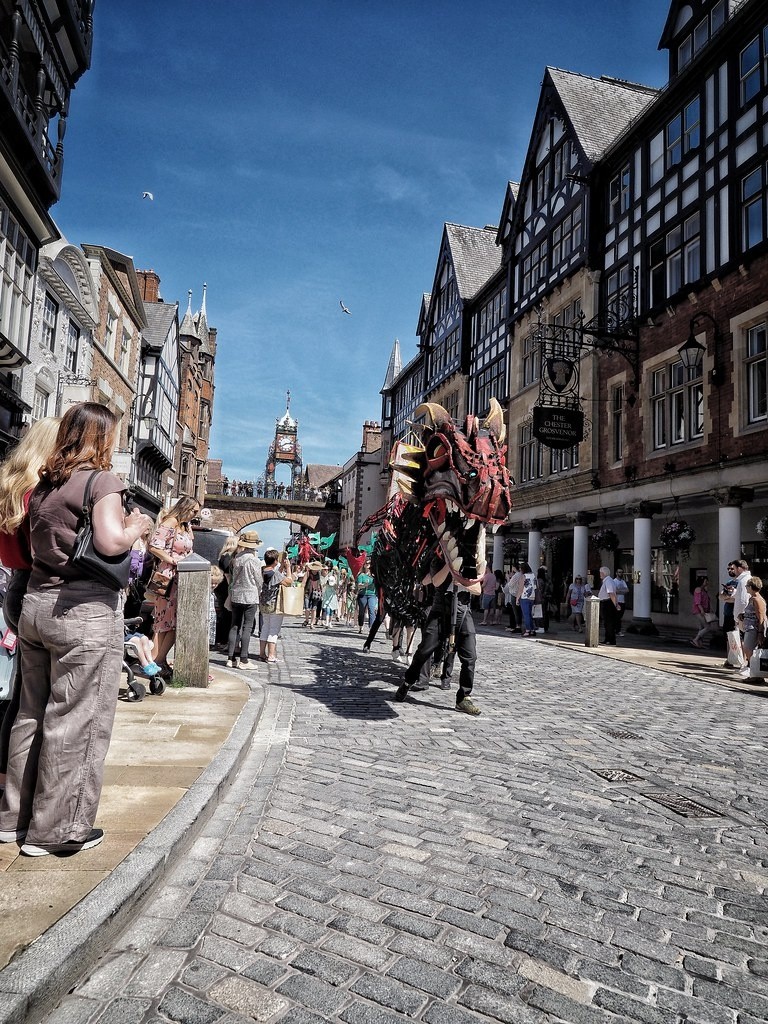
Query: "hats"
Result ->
[[341, 568, 347, 574], [237, 530, 263, 549], [309, 563, 321, 570]]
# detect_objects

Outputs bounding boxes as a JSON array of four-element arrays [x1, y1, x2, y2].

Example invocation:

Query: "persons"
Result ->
[[0, 401, 161, 857], [741, 575, 768, 664], [719, 559, 752, 669], [208, 565, 224, 680], [615, 568, 629, 637], [478, 561, 553, 637], [148, 495, 203, 679], [216, 477, 330, 504], [690, 576, 712, 648], [650, 579, 679, 614], [559, 575, 570, 623], [598, 566, 622, 645], [564, 575, 591, 634], [282, 549, 415, 662], [218, 530, 293, 669], [395, 555, 482, 716]]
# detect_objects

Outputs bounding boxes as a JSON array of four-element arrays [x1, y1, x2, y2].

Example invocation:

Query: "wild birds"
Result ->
[[142, 192, 153, 201], [339, 300, 351, 314]]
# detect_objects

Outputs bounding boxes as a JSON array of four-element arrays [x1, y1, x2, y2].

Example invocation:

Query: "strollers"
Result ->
[[119, 615, 166, 702]]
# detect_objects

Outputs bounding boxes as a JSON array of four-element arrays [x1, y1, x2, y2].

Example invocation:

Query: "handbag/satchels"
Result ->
[[532, 604, 543, 618], [727, 626, 744, 665], [147, 572, 173, 598], [750, 645, 768, 678], [704, 612, 719, 622], [570, 599, 577, 606], [73, 469, 131, 592], [312, 590, 324, 600]]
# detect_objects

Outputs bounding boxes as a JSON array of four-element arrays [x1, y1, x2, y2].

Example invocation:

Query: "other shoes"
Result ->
[[474, 610, 768, 686], [411, 679, 429, 690], [141, 607, 482, 677], [21, 828, 105, 856], [441, 680, 451, 690], [0, 826, 28, 843]]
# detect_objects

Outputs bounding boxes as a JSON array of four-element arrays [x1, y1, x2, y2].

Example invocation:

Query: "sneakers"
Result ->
[[395, 681, 411, 702], [455, 696, 481, 715]]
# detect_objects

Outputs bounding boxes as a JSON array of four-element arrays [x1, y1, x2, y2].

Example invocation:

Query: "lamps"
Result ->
[[678, 311, 724, 386], [130, 394, 159, 430]]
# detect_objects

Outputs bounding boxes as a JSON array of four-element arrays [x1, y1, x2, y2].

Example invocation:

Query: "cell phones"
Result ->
[[721, 583, 728, 589], [283, 551, 288, 560]]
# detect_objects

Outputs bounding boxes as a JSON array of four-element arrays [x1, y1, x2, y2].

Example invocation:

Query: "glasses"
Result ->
[[726, 567, 734, 571], [192, 508, 198, 515], [575, 577, 582, 581]]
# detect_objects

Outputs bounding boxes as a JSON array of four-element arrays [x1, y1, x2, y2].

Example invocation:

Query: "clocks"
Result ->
[[277, 435, 294, 452]]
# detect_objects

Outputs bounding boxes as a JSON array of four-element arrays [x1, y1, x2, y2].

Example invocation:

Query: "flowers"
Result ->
[[501, 537, 522, 560], [751, 512, 768, 545], [657, 520, 696, 570], [591, 529, 620, 556], [539, 536, 562, 562]]
